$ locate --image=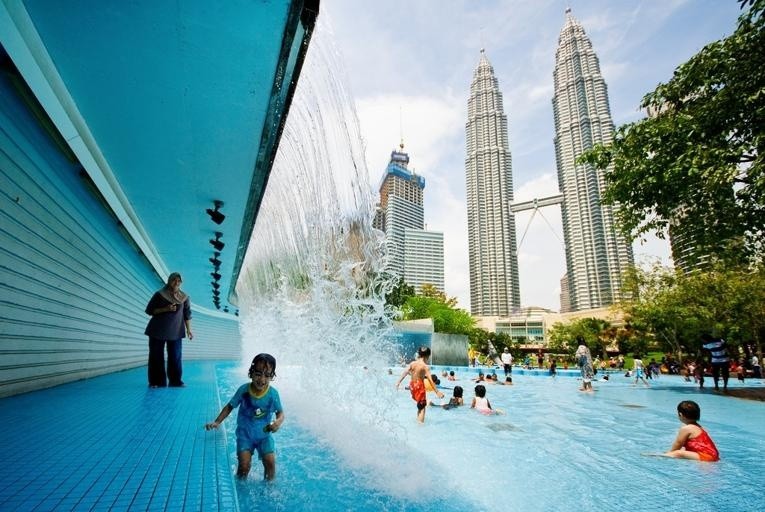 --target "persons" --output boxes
[203,352,285,482]
[402,369,507,418]
[143,271,194,388]
[469,346,765,387]
[641,400,720,462]
[696,333,740,393]
[396,346,443,423]
[574,334,594,393]
